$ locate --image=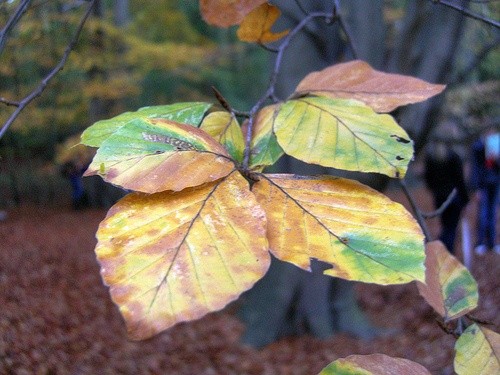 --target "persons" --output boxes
[421,138,468,253]
[468,122,500,256]
[59,162,117,211]
